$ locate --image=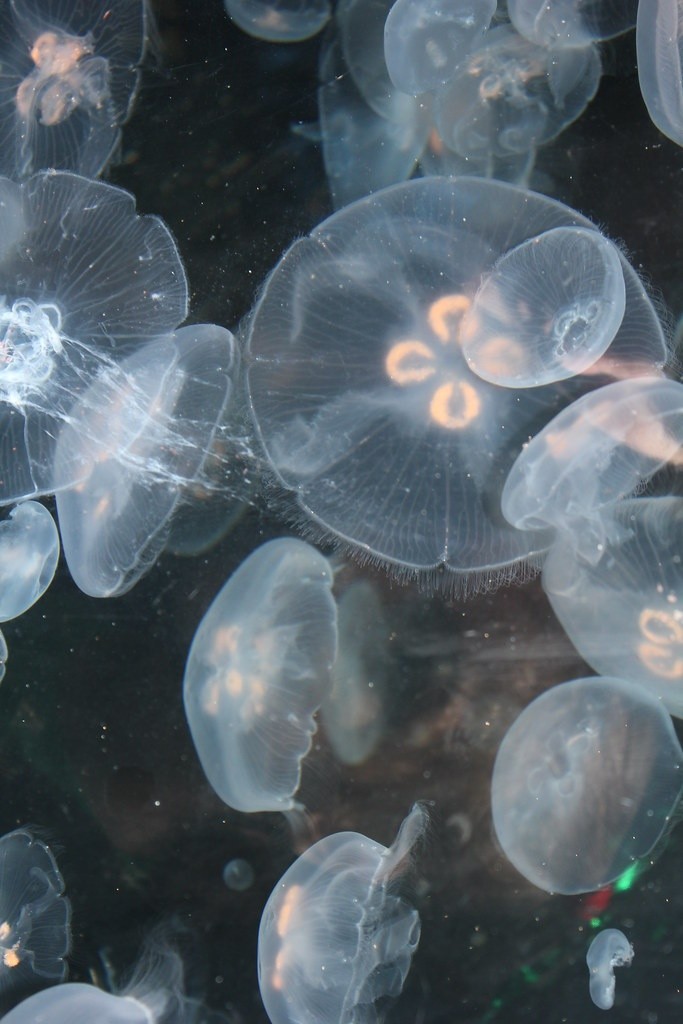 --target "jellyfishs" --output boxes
[4,0,679,1023]
